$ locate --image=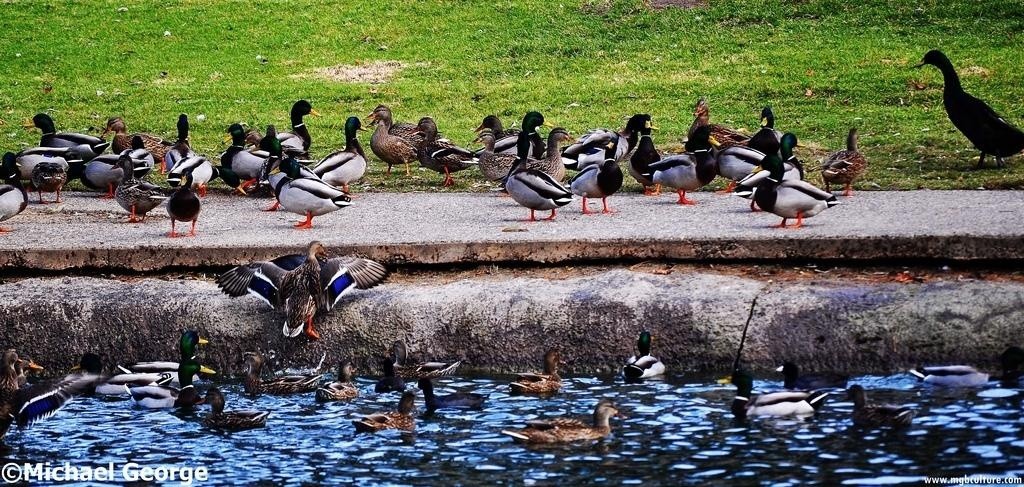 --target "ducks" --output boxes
[748,105,782,155]
[352,393,420,429]
[473,128,516,194]
[390,340,459,378]
[506,131,572,221]
[216,241,390,339]
[717,373,827,423]
[316,358,363,399]
[912,50,1024,169]
[837,383,917,432]
[461,111,554,164]
[119,137,154,177]
[260,124,282,161]
[103,115,174,165]
[774,363,799,389]
[419,375,488,407]
[571,136,628,215]
[622,332,666,379]
[527,127,576,183]
[627,114,662,196]
[0,152,29,231]
[732,132,804,211]
[314,116,367,196]
[912,345,1023,391]
[111,154,163,223]
[474,115,521,140]
[239,350,322,394]
[126,361,217,409]
[410,115,471,188]
[822,127,866,196]
[109,331,209,382]
[194,389,270,431]
[14,354,44,388]
[72,353,174,394]
[165,114,197,171]
[221,123,265,193]
[751,153,841,230]
[245,129,312,167]
[368,105,418,177]
[166,168,201,238]
[13,353,107,427]
[25,113,110,167]
[561,111,640,171]
[0,147,83,180]
[716,145,766,193]
[268,99,321,159]
[0,349,108,440]
[365,118,441,146]
[168,156,247,198]
[249,135,321,211]
[70,149,134,199]
[685,97,752,150]
[374,358,407,391]
[641,125,721,205]
[510,349,567,396]
[31,162,69,203]
[501,400,624,444]
[269,158,353,228]
